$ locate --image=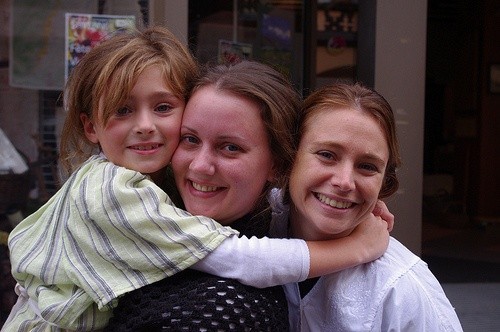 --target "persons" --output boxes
[0,27,395,332]
[104,56,304,332]
[265,82,464,332]
